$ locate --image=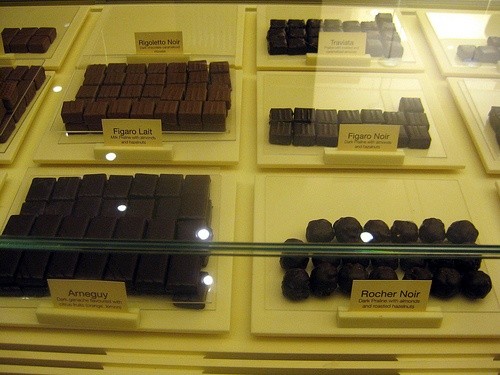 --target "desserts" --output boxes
[0,13,500,309]
